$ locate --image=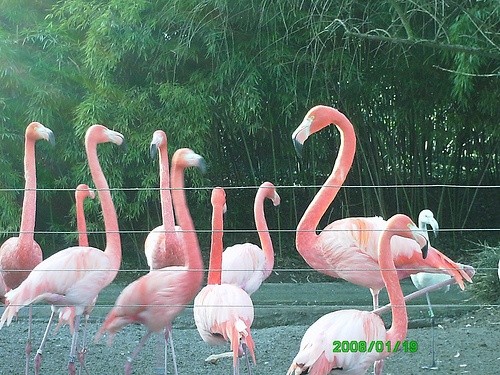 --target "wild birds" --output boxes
[0,121,282,375]
[406,208,475,319]
[287,213,428,375]
[292,104,472,323]
